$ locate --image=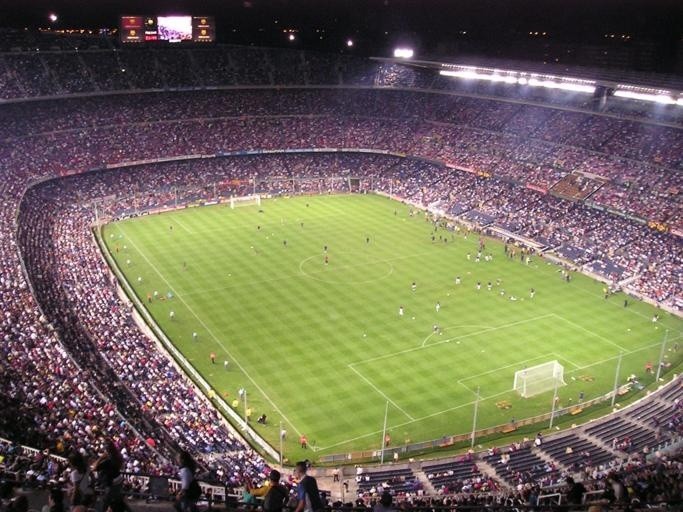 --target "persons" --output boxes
[398,304,404,316]
[300,222,303,228]
[324,254,328,264]
[277,373,683,512]
[432,321,439,335]
[0,208,277,512]
[651,314,658,323]
[644,360,652,374]
[365,237,369,244]
[409,207,682,315]
[282,239,286,248]
[0,26,683,208]
[659,362,673,367]
[323,246,326,252]
[673,341,679,354]
[394,209,396,216]
